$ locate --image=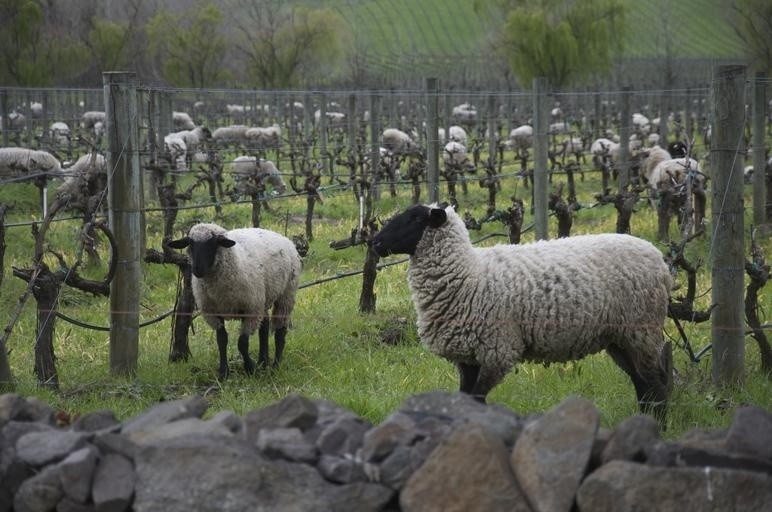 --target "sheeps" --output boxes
[166,220,303,377]
[373,195,676,434]
[0,101,772,232]
